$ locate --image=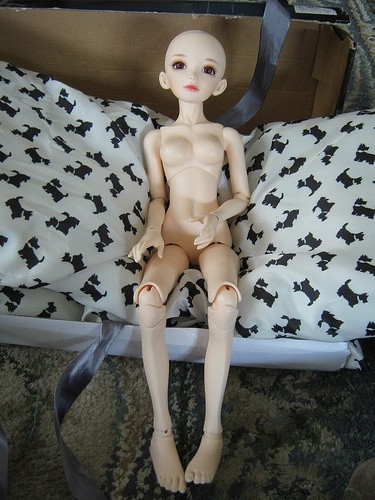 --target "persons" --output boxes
[130,30,251,494]
